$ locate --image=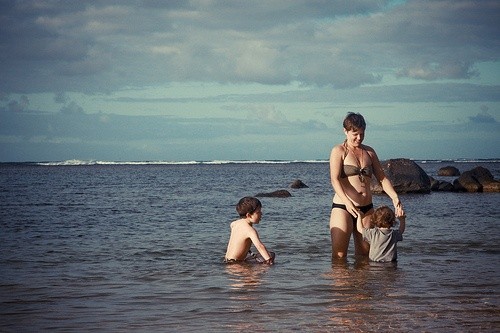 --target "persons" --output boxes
[329,112,407,265]
[223,196,274,265]
